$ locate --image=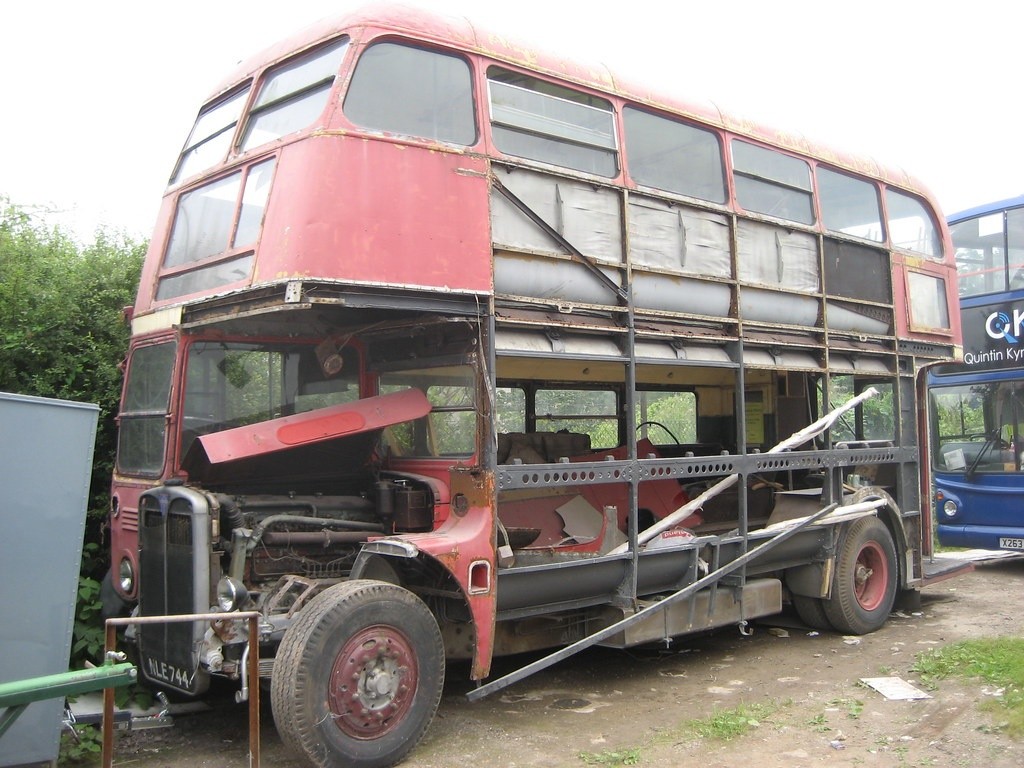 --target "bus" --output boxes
[97,1,965,768]
[931,188,1023,557]
